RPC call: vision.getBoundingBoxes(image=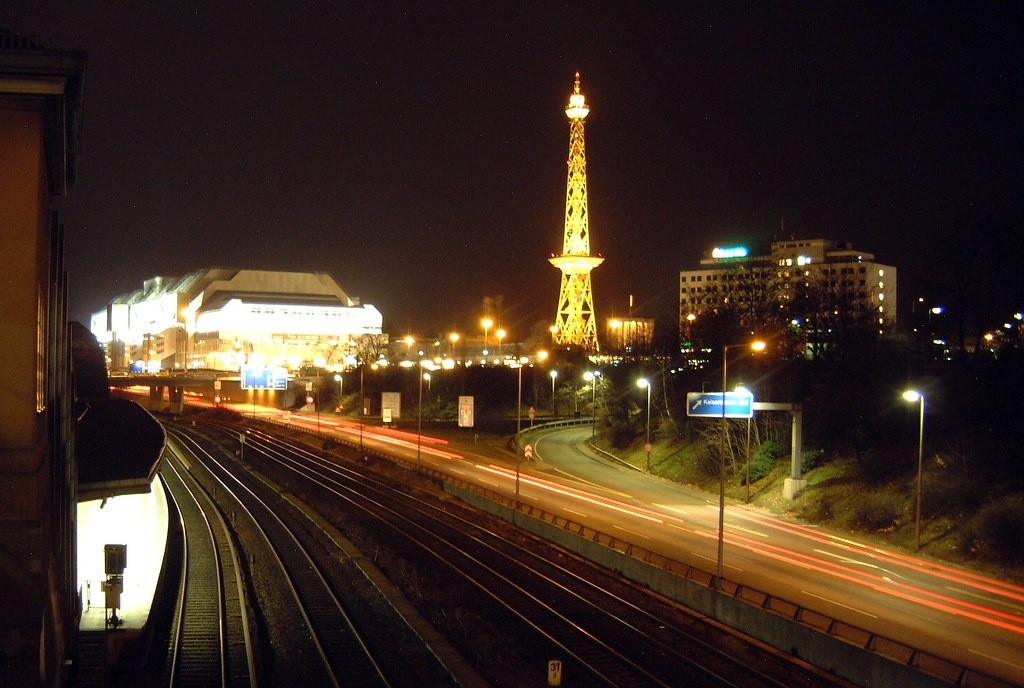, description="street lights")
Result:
[714,340,767,579]
[334,375,343,399]
[550,370,559,415]
[901,389,925,554]
[635,377,656,474]
[483,318,492,352]
[583,371,596,437]
[497,329,504,355]
